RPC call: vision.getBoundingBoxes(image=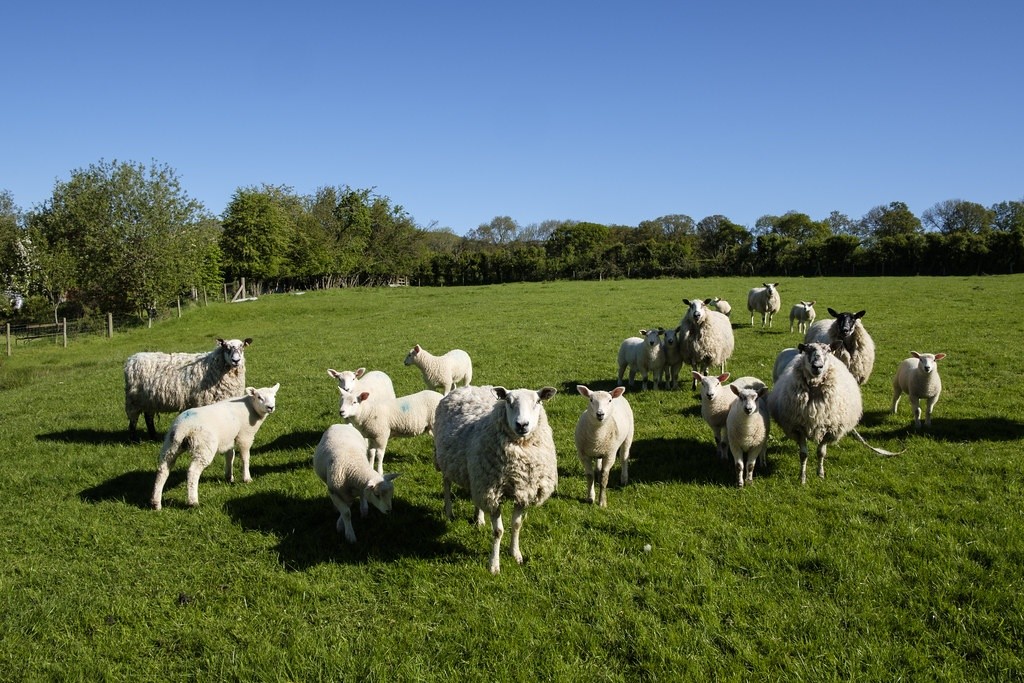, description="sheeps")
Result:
[119,282,947,571]
